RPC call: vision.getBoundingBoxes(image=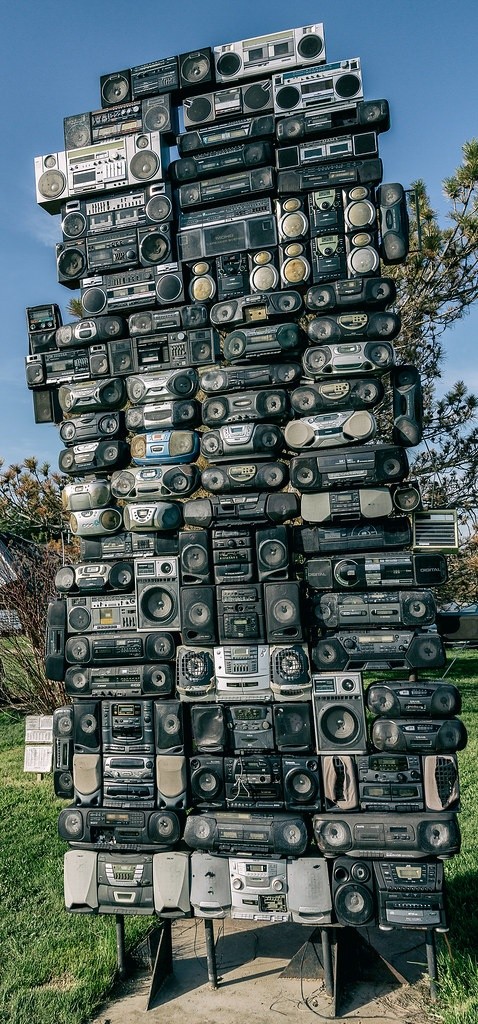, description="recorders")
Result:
[25,23,470,928]
